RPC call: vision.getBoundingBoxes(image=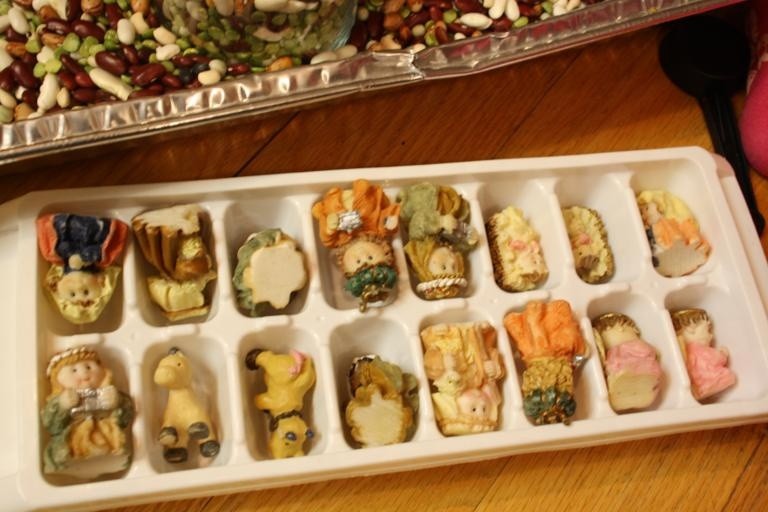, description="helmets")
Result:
[0,145,768,512]
